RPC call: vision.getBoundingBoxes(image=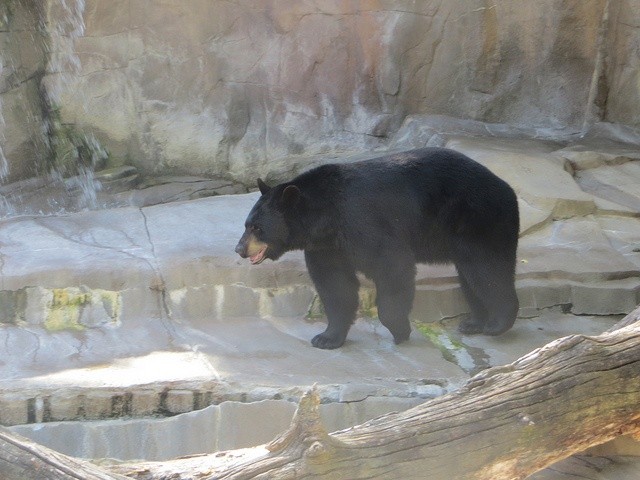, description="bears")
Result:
[234,148,520,351]
